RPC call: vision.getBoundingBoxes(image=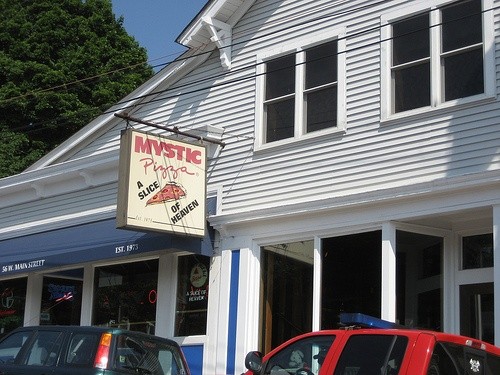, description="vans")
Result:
[240,313,500,375]
[0,324,190,375]
